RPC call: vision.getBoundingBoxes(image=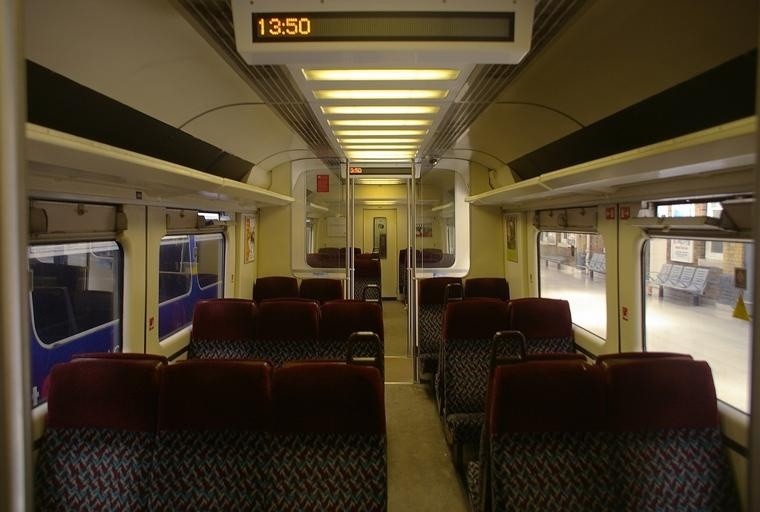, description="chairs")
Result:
[305,243,443,299]
